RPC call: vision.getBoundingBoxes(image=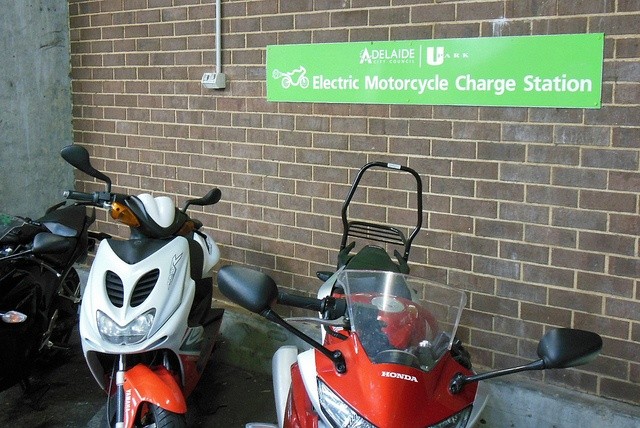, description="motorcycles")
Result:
[217,162,602,428]
[61,145,224,428]
[0,202,112,411]
